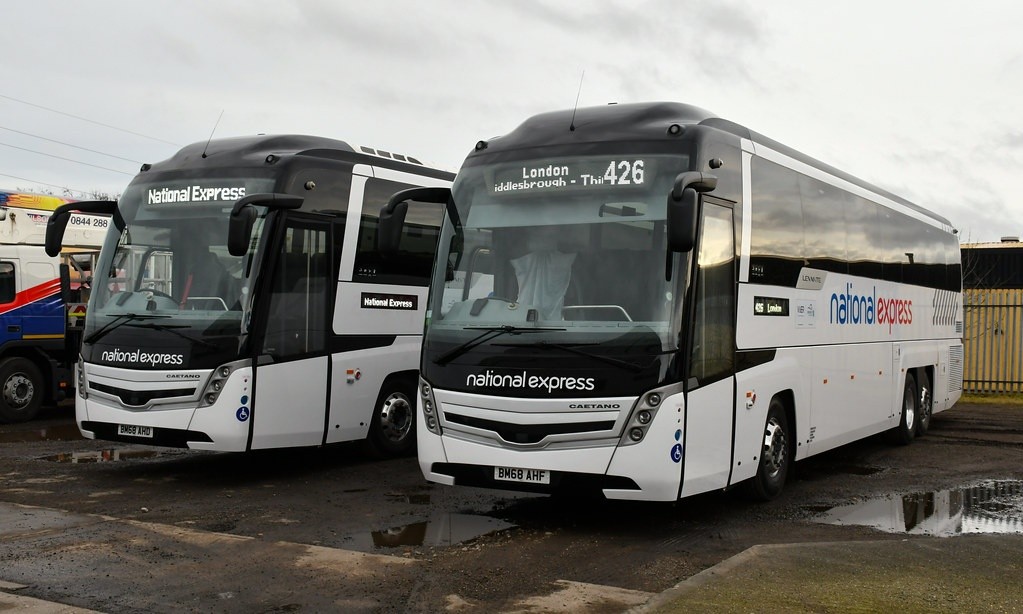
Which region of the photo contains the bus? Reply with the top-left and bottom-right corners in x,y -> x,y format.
0,186 -> 248,431
958,235 -> 1023,392
376,100 -> 962,518
48,131 -> 494,458
